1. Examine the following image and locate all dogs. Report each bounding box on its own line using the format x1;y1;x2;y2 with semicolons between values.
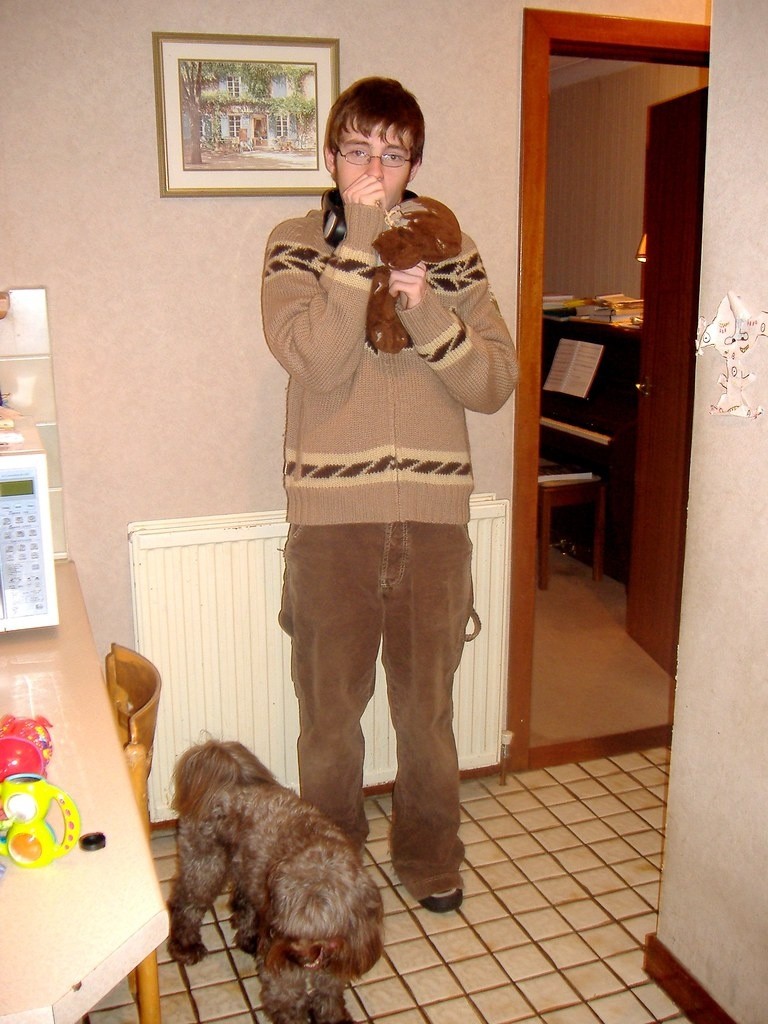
165;737;384;1024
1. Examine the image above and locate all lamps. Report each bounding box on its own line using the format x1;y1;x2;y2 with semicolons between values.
635;233;647;263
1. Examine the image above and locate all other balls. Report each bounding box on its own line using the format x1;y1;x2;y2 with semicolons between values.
0;719;53;784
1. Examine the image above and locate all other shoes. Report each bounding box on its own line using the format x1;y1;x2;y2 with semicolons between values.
421;887;461;911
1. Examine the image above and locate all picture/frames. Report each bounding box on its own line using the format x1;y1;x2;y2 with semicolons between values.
152;30;341;198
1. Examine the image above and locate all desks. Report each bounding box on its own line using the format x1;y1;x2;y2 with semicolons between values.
0;558;172;1024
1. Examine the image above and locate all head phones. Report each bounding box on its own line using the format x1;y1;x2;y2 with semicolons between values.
322;190;418;247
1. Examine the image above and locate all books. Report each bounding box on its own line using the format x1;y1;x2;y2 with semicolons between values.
543;338;603;398
543;293;645;323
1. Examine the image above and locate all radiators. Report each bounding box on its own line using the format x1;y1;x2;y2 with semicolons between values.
127;494;509;827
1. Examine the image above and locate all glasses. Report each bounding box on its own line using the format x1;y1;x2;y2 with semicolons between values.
336;147;416;166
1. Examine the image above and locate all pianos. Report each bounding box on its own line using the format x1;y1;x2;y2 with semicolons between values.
541;317;641;585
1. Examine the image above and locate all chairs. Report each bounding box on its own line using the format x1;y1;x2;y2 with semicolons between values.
104;641;163;1024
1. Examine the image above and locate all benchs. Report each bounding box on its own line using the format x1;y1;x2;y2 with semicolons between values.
538;457;605;590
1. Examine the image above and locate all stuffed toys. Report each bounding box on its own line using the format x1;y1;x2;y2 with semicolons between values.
367;196;463;354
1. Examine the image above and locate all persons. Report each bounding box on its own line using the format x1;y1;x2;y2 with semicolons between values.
261;77;518;912
